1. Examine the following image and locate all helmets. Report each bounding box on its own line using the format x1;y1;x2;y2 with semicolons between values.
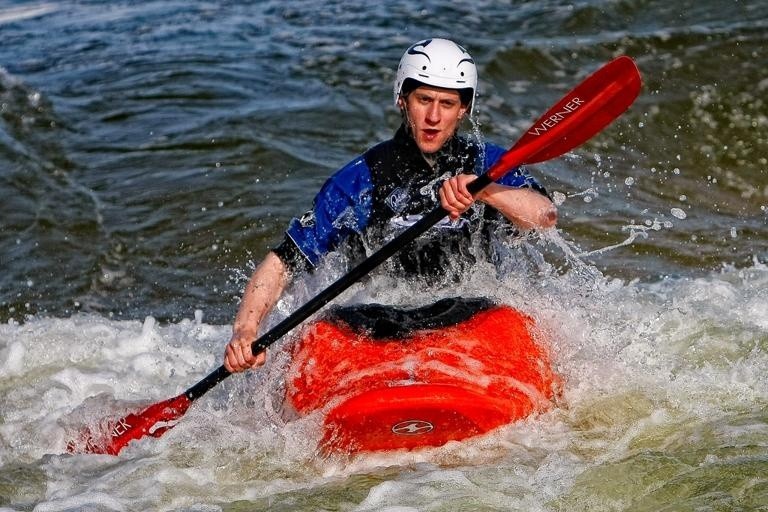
395;38;478;117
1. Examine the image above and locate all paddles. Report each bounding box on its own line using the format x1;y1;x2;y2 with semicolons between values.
67;53;642;455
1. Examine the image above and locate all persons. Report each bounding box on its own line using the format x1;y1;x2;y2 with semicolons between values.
223;37;562;377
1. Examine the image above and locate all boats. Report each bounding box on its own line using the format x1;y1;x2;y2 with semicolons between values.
282;295;566;463
